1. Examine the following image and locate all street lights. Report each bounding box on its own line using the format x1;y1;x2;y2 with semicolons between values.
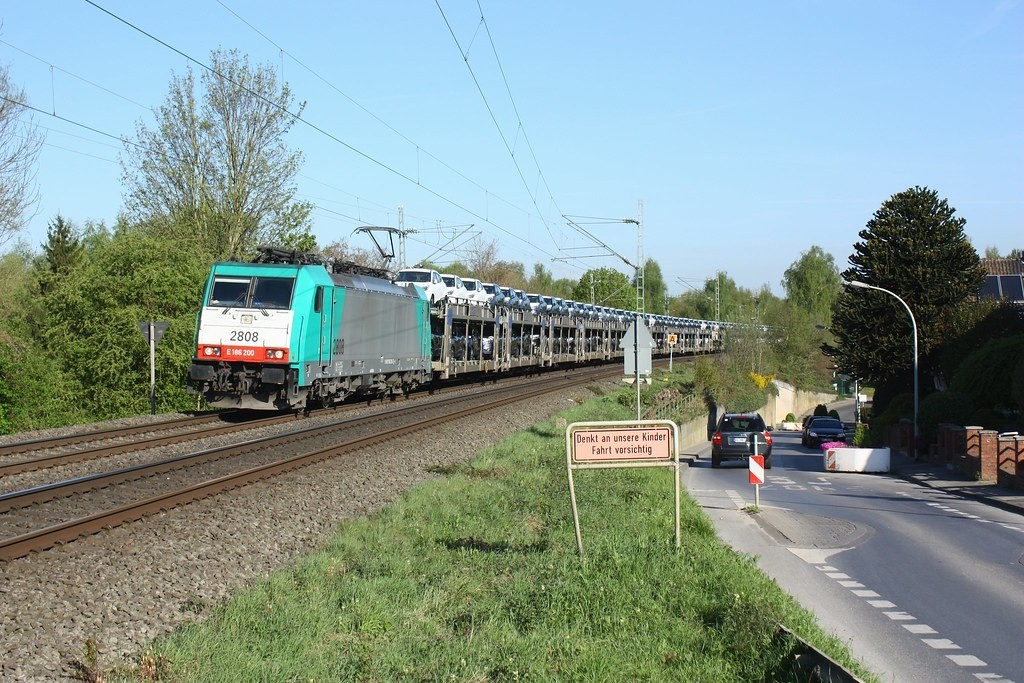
849;280;918;462
815;324;860;424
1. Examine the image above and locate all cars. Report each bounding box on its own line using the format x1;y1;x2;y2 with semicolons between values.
801;416;834;445
805;419;846;449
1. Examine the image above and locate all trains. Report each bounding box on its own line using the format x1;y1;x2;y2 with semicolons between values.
190;246;781;415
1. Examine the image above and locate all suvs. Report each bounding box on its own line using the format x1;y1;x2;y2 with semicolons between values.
709;412;773;469
